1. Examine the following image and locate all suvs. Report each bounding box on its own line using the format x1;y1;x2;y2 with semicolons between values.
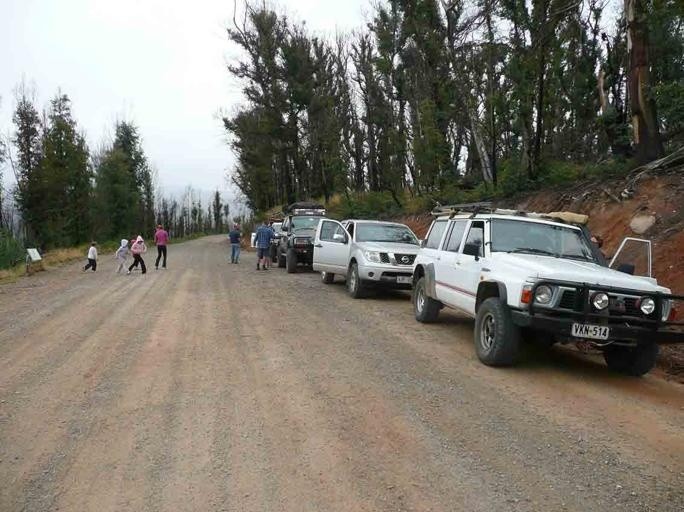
412;202;684;376
267;214;331;272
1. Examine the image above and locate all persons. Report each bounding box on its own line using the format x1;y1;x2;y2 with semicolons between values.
253;220;281;270
115;235;147;274
153;225;169;270
83;241;99;272
590;234;604;247
228;223;242;264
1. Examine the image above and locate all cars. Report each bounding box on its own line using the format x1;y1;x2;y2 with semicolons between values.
313;219;420;300
